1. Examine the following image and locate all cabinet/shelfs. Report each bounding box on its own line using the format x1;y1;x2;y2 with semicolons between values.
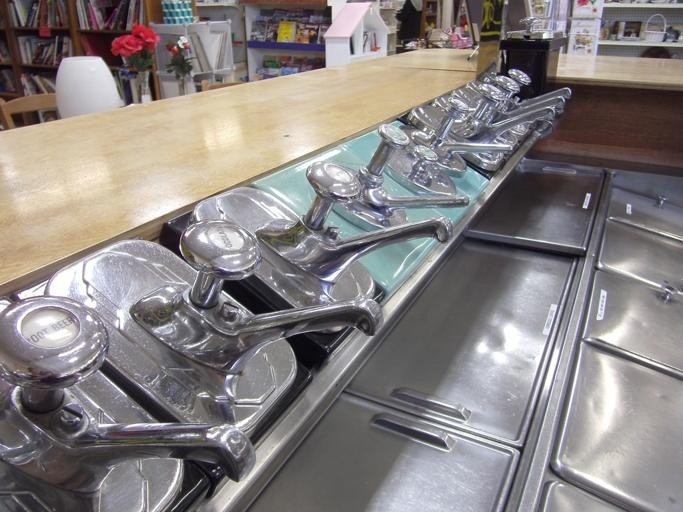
596;0;683;59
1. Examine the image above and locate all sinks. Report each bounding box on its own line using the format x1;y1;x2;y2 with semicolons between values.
506;29;564;38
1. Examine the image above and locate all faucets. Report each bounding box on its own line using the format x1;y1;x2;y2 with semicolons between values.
391;70;572;207
519;15;551;32
192;161;454;364
2;296;255;511
41;218;384;482
321;123;471;243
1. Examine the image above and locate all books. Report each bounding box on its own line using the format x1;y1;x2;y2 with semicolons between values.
253;53;323;79
249;7;329;44
0;0;145;123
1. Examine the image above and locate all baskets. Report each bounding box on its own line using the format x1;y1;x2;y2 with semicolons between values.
645;14;667;44
